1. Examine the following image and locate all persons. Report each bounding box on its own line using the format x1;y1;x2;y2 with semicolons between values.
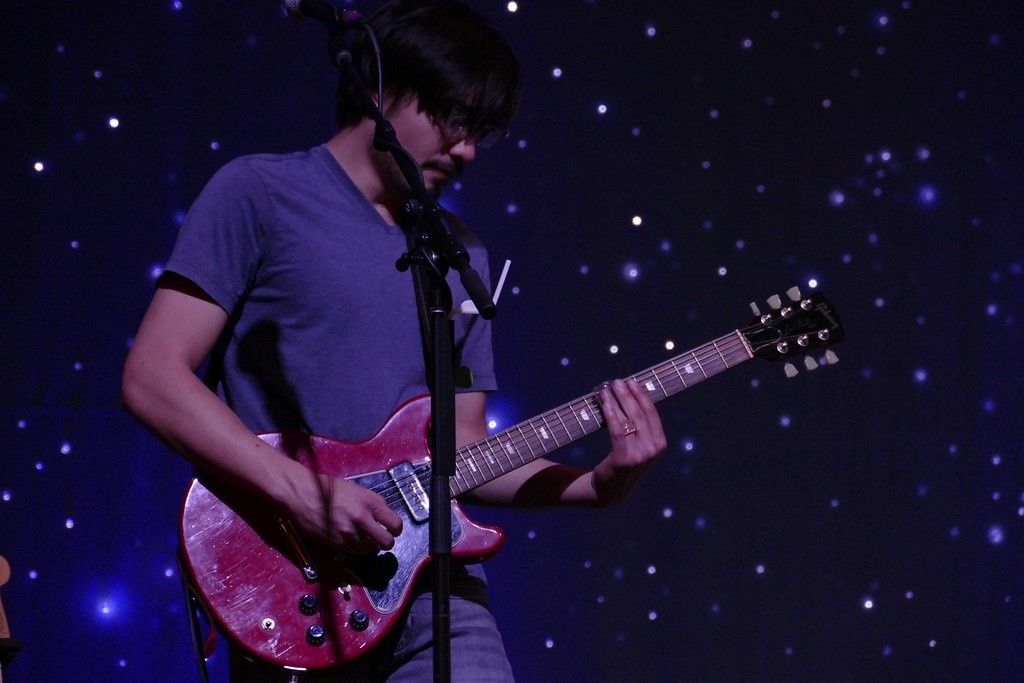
120;0;671;682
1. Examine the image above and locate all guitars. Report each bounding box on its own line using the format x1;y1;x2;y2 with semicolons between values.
168;283;858;678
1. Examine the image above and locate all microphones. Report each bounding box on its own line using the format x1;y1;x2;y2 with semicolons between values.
284;0;371;29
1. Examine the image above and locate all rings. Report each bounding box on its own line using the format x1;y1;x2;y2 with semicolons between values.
623;416;637;436
